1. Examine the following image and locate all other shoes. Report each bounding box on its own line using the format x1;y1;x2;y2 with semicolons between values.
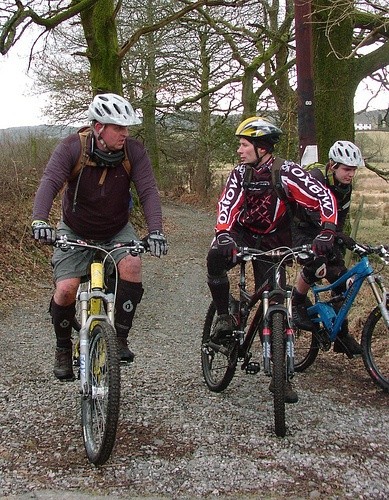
333;333;363;354
212;314;234;339
291;296;314;331
268;375;299;404
53;345;74;380
115;337;135;364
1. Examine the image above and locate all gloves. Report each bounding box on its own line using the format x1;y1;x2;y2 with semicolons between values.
146;230;168;258
214;233;239;264
29;220;56;247
334;232;356;251
311;230;335;256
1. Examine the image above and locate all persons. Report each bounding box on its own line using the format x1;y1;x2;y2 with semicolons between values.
203;117;337;404
32;93;170;380
278;138;369;355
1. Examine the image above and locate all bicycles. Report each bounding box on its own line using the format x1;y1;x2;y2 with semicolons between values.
258;238;389;393
201;243;318;438
32;233;171;464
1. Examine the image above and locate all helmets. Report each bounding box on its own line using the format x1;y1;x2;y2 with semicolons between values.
329;140;365;168
87;92;142;127
234;116;283;144
